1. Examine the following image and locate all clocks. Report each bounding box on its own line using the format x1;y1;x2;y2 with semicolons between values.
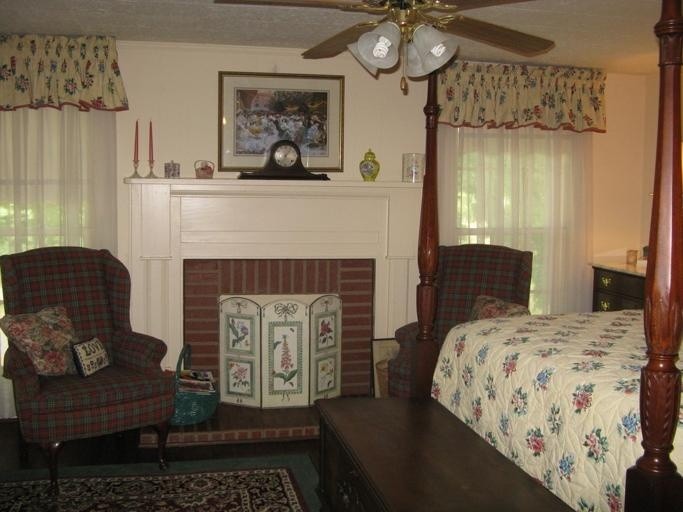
238;141;331;179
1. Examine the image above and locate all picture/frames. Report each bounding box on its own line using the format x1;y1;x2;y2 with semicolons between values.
217;70;345;175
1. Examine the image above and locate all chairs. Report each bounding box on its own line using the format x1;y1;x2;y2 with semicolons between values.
385;241;534;400
0;246;179;511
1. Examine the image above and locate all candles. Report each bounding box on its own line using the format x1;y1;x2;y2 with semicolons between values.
133;118;139;161
147;120;154;160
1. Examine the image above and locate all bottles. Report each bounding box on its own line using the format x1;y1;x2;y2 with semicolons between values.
360;147;379;182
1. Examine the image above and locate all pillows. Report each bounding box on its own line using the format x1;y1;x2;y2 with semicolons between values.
0;307;78;378
70;335;112;378
471;293;530;321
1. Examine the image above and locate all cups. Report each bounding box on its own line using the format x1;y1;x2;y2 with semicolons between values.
163;160;180;178
625;264;636;273
402;152;425;182
625;250;638;265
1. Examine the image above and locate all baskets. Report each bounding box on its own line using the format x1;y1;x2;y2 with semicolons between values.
169;344;219;426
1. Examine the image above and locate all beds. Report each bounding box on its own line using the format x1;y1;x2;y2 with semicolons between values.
411;0;683;512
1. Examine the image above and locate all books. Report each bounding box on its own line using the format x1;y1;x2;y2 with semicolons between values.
179;370;215;393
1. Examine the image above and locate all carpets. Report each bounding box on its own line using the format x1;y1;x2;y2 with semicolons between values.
4;450;318;512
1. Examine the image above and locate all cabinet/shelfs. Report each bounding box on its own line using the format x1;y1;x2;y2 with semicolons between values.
590;255;647;310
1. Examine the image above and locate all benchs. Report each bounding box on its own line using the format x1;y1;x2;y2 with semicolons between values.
313;396;580;511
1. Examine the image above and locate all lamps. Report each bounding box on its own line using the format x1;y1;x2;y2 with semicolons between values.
347;21;460;81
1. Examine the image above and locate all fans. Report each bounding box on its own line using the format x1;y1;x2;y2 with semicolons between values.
210;0;556;59
